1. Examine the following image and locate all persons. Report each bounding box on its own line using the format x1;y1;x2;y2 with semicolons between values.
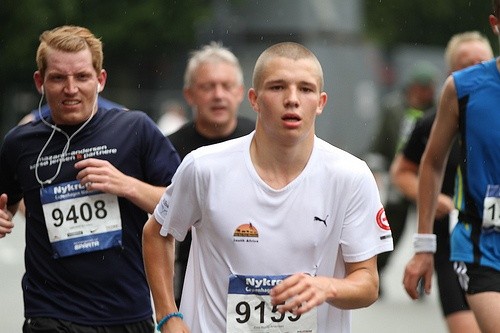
141;42;394;333
0;26;256;333
371;7;500;333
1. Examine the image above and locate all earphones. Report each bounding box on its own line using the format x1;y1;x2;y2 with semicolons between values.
40;84;44;95
97;82;101;93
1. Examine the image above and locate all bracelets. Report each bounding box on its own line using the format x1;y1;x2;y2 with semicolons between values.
413;234;437;253
156;313;184;330
